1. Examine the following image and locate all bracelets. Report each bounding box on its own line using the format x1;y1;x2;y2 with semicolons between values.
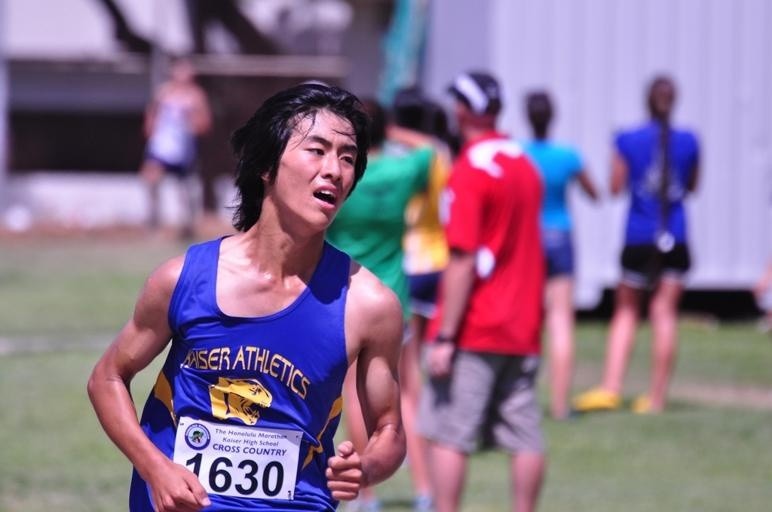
433;333;458;344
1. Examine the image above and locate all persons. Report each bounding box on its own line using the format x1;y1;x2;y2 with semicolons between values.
319;69;469;510
139;54;210;243
570;75;701;415
420;65;553;511
510;88;601;421
88;77;410;511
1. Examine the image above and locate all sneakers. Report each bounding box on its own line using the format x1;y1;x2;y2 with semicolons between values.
632;396;652;414
570;390;618;414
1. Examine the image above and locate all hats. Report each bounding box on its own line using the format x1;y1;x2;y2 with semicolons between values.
448;73;500;113
